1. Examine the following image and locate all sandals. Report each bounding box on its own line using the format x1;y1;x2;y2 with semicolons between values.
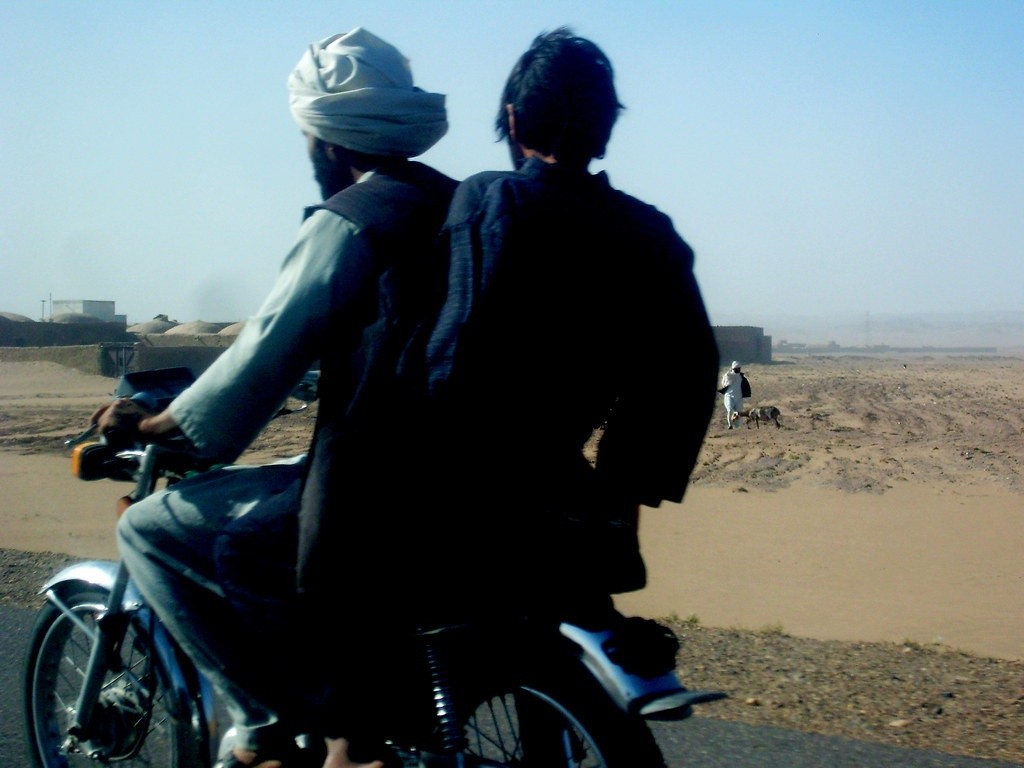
212;745;287;767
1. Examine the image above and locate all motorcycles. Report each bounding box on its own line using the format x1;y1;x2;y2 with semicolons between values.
22;364;727;768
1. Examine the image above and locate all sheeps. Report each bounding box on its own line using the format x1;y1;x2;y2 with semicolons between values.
731;405;782;429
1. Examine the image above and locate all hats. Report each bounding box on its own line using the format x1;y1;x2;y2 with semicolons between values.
732;360;742;369
286;27;448;158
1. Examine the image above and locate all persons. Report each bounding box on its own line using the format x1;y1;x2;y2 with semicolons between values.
722;360;743;429
96;25;723;768
